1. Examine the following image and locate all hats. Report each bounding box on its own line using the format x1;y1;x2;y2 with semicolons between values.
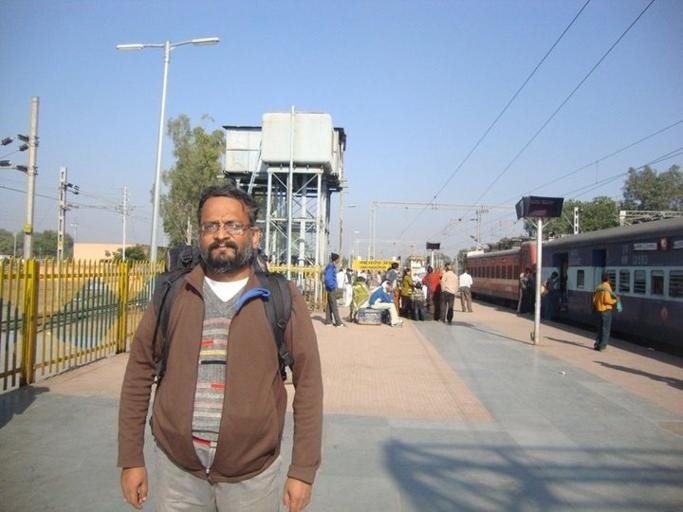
331;253;339;262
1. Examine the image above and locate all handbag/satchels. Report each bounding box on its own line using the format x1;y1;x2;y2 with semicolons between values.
540;286;548;295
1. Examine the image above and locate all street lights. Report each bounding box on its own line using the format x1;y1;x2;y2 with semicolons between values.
69;221;78;240
112;33;221;262
338;203;357;265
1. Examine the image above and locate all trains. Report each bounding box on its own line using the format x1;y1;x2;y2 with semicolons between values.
458;215;682;345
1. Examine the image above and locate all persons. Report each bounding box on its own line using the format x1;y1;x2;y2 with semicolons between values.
117;183;323;512
322;252;474;330
516;266;568;322
590;273;623;354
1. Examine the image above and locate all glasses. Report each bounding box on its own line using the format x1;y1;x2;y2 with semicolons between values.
200;223;252;235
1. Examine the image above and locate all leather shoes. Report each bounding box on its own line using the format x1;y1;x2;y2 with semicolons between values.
600;348;611;353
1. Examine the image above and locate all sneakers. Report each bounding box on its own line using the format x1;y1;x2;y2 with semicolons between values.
392;320;403;326
336;323;347;328
